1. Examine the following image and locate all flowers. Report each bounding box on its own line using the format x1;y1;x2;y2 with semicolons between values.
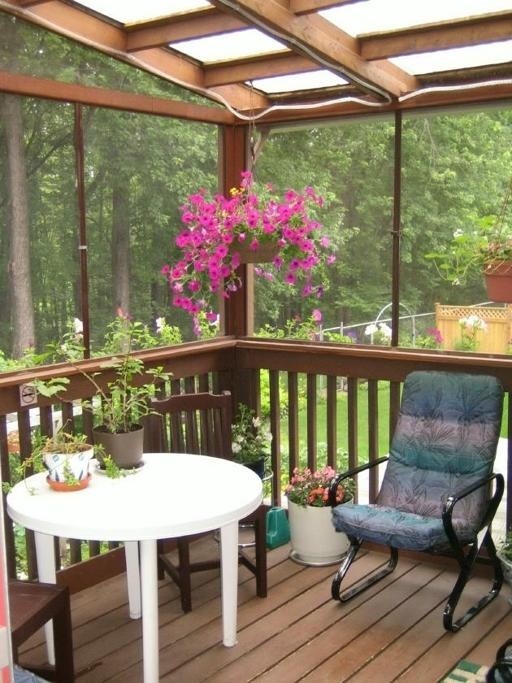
225;404;273;461
58;302;180;362
162;169;336;340
425;220;512;286
418;325;443;346
285;465;355;507
451;313;487;351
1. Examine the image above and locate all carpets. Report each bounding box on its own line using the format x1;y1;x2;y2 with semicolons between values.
444;661;490;683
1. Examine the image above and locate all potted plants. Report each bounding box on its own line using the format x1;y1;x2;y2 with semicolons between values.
1;418;126;497
33;320;173;467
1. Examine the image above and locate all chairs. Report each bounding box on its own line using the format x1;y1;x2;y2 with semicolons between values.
4;493;76;683
149;391;270;614
6;453;263;683
328;371;505;634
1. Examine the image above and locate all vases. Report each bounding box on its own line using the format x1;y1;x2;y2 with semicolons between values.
288;496;354;561
225;455;264;481
228;238;282;263
483;260;512;304
